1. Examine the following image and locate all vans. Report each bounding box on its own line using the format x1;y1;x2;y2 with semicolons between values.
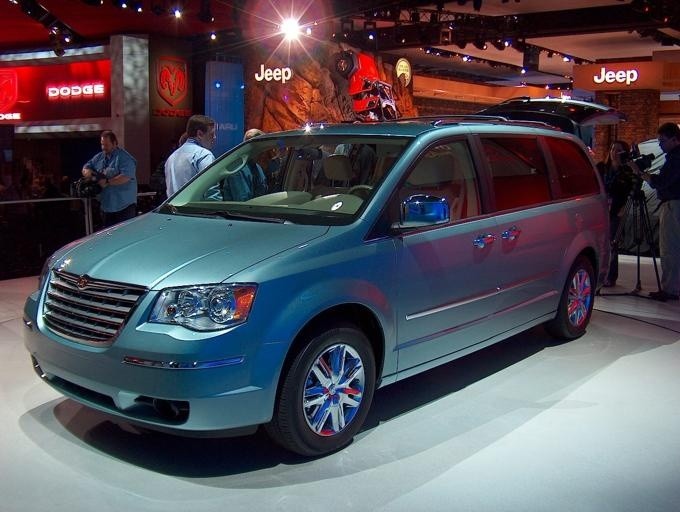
19;94;631;457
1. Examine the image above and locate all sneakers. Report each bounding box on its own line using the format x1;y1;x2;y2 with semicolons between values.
604;279;616;287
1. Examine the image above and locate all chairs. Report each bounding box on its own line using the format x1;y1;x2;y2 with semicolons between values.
315;154;404;196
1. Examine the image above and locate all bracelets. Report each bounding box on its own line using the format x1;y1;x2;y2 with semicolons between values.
106;178;110;183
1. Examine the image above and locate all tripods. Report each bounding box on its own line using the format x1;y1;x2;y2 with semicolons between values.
82;198;95;235
596;180;665;302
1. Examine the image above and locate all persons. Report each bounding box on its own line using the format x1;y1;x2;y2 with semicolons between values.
596;123;680;300
81;131;137;228
150;114;378;209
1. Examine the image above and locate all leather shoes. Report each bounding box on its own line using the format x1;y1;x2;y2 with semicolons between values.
649;291;679;302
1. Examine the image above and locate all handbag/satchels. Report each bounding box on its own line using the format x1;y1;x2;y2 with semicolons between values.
70;175;109;198
